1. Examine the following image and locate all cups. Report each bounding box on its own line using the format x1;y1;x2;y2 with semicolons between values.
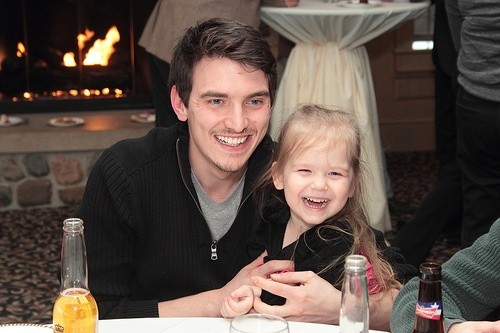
229;313;289;333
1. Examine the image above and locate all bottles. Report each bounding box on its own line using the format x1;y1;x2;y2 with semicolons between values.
413;262;444;333
52;218;99;332
339;255;369;333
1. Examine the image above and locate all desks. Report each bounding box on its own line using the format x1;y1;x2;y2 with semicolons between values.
257;1;431;232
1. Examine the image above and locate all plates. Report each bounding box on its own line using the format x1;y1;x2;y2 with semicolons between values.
48;117;84;126
1;116;22;127
131;112;156;123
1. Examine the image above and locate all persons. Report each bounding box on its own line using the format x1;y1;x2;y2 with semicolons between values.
389;217;500;333
137;0;263;127
221;106;404;318
81;18;421;333
456;0;500;249
